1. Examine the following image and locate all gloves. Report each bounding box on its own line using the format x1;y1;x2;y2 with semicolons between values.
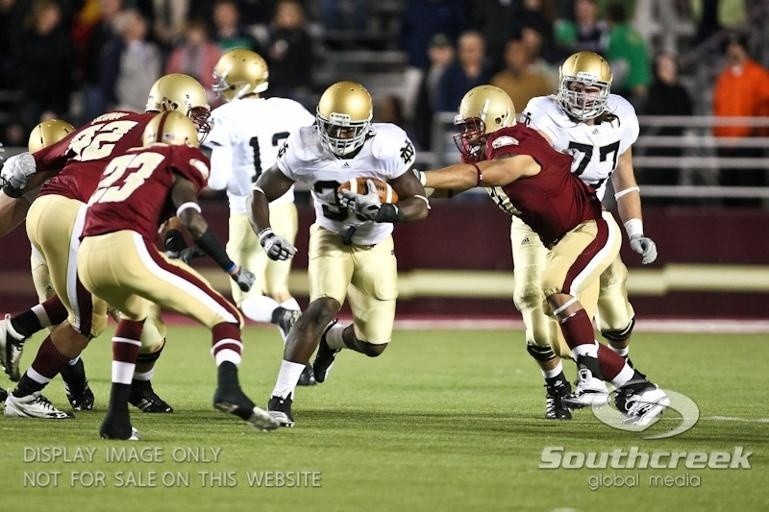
232;264;256;291
258;228;297;261
1;153;36;191
338;180;387;223
628;233;658;264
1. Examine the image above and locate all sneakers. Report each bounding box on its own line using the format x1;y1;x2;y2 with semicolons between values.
213;381;279;430
131;380;172;413
268;307;339;427
544;355;672;426
1;314;93;422
100;424;141;442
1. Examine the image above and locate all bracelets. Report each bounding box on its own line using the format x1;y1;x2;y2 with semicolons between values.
623;217;644;238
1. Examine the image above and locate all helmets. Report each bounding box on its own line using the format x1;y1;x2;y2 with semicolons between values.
452;84;516;143
558;51;613;106
316;81;373;151
27;119;74;154
211;48;268;101
143;73;209;146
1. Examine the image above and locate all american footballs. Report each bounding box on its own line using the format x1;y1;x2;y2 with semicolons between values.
336;176;400;207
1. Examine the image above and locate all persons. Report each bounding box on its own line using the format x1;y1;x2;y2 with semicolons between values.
0;75;211;420
510;50;657;421
245;80;430;427
75;110;280;441
0;119;173;414
197;50;316;385
408;86;669;421
1;0;769;210
